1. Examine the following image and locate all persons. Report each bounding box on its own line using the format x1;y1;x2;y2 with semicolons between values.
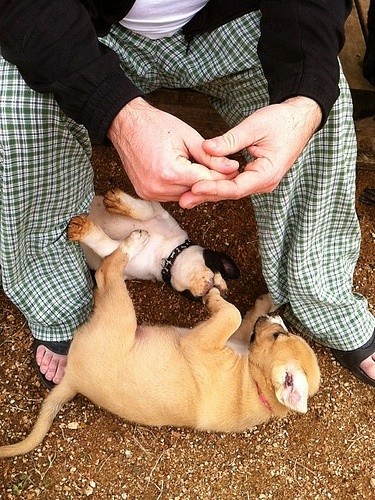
1;1;375;386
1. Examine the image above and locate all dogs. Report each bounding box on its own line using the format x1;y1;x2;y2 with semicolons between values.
1;230;321;459
68;186;242;305
